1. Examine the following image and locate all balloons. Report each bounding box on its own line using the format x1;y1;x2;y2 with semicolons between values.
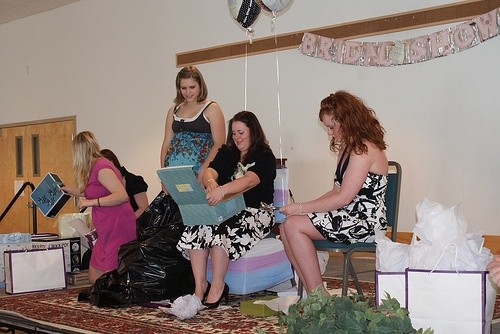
228;0;290;28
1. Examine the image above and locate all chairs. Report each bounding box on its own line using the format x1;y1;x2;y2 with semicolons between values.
297;161;402;304
206;158;297;299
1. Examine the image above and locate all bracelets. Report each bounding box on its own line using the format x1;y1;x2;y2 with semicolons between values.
97;198;101;207
205;179;215;185
299;202;304;215
220;185;225;197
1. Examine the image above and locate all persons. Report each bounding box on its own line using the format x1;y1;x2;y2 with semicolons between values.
100;149;148;219
487;255;500;287
279;92;389;313
176;111;277;308
56;132;136;284
161;66;226;196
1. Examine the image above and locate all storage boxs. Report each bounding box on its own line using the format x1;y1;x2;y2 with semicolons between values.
30;174;70;219
157;165;245;226
32;237;82;274
241;297;280;317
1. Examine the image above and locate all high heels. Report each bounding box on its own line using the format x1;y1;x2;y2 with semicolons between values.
199;281;211;303
203;282;229;310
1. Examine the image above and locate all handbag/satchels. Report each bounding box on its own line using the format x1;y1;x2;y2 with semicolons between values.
374;269;406;311
404;242;498;334
3;246;66;294
